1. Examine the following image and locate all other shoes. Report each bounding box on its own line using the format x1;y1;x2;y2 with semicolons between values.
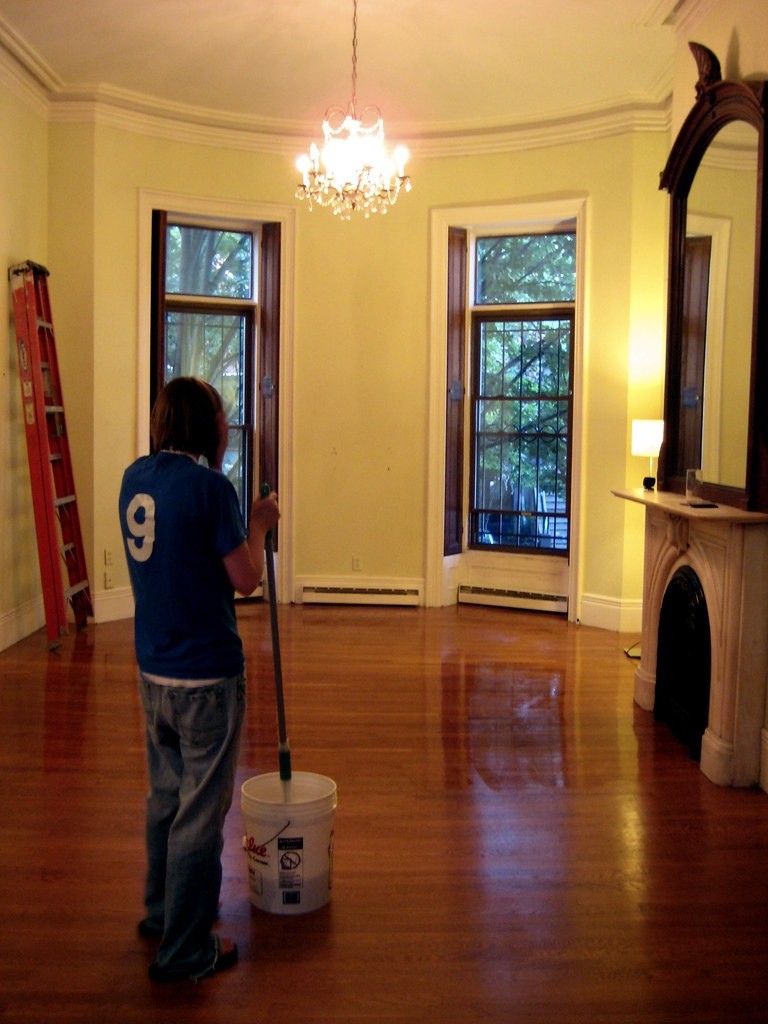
214;938;239;975
138;920;159;939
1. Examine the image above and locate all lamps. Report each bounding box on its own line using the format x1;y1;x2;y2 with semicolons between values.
631;420;664;490
296;0;412;222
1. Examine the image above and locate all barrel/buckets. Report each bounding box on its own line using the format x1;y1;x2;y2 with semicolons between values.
239;770;338;915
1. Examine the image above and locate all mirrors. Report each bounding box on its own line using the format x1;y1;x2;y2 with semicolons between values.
656;40;768;511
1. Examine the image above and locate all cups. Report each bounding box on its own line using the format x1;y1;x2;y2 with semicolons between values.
686;469;703;505
643;477;655;490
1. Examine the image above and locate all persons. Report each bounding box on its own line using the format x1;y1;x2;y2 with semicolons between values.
117;377;283;987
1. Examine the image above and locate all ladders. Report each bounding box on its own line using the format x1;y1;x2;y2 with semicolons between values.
9;259;97;651
